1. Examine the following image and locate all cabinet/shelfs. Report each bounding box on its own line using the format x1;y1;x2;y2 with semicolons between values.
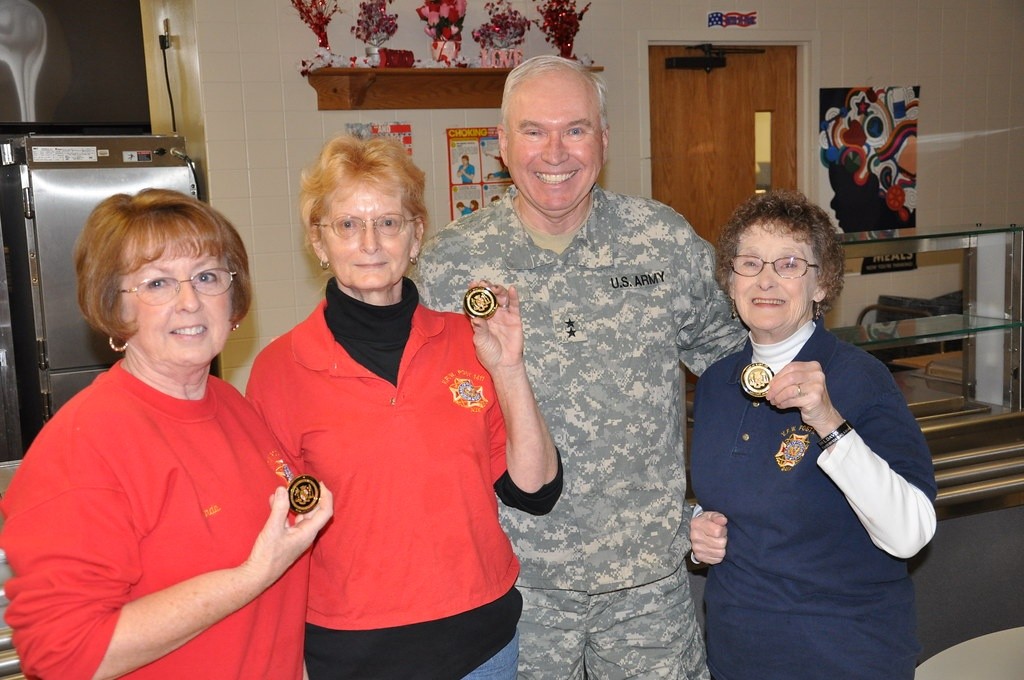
827;224;1023;420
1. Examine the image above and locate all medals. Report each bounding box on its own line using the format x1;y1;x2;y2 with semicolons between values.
741;362;775;398
287;474;320;514
464;287;498;322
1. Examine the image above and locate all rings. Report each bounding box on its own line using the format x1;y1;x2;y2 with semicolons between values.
497;304;508;308
798;384;801;395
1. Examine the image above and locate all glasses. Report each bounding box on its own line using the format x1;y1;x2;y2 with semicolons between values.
313;214;420;239
729;255;820;280
118;268;236;306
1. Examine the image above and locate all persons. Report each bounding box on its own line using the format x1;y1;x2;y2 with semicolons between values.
406;56;748;680
244;137;564;679
1;188;334;680
686;193;938;680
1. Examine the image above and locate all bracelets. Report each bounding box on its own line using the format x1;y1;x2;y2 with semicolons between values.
816;420;854;451
